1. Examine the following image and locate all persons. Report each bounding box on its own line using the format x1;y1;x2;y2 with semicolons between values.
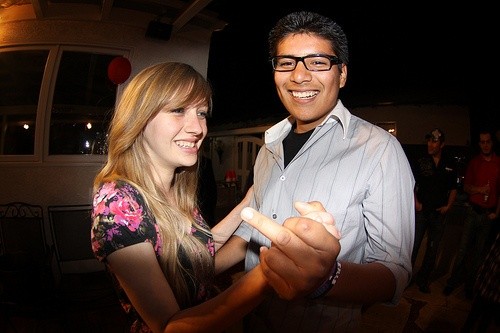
411;128;458;293
442;129;500;299
212;11;416;333
90;62;343;333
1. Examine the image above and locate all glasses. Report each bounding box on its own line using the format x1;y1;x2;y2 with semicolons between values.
269;53;341;72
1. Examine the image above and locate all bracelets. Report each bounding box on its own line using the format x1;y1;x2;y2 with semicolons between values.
316;262;341;296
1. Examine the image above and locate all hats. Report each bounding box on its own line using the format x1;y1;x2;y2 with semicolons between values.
425;127;446;142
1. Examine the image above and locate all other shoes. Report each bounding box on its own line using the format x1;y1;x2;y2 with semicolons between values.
415;273;430;294
443;284;454;296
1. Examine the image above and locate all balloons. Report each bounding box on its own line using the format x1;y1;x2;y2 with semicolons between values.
107;57;132;85
